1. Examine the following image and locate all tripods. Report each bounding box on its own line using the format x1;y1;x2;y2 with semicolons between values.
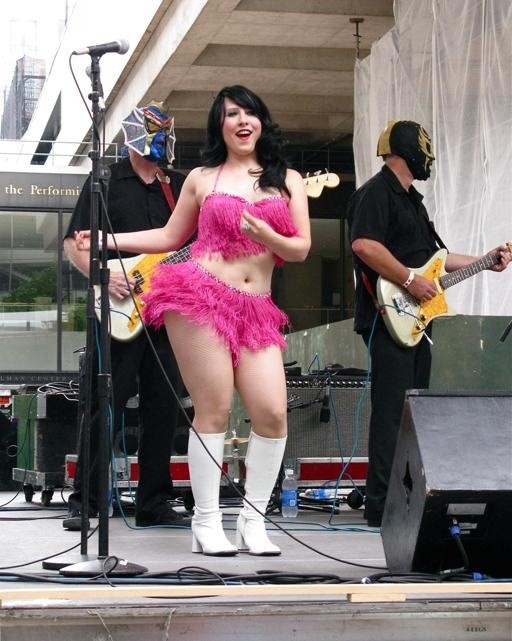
265;461;340;517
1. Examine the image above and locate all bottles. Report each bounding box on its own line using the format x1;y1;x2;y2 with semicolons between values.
313;489;332;498
97;471;114;517
281;469;299;518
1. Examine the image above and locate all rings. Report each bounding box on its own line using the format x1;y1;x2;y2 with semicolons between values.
242;223;249;232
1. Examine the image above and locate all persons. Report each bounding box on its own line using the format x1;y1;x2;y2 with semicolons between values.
73;85;312;556
344;122;511;527
63;105;192;529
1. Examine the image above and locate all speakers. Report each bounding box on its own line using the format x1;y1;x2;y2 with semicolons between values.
281;375;372;458
380;386;512;580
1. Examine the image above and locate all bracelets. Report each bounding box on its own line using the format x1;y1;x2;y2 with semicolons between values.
402;271;415;288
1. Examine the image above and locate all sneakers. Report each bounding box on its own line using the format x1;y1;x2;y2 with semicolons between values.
63;510;89;531
136;507;192;526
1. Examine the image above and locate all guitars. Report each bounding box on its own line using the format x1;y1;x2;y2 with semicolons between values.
377;240;512;348
94;168;341;343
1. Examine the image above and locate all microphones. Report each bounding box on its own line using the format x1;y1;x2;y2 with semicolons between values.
85;66;106;112
72;38;130;55
320;378;331;423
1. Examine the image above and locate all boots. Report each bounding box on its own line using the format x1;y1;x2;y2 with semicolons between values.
236;428;287;556
187;428;238;555
